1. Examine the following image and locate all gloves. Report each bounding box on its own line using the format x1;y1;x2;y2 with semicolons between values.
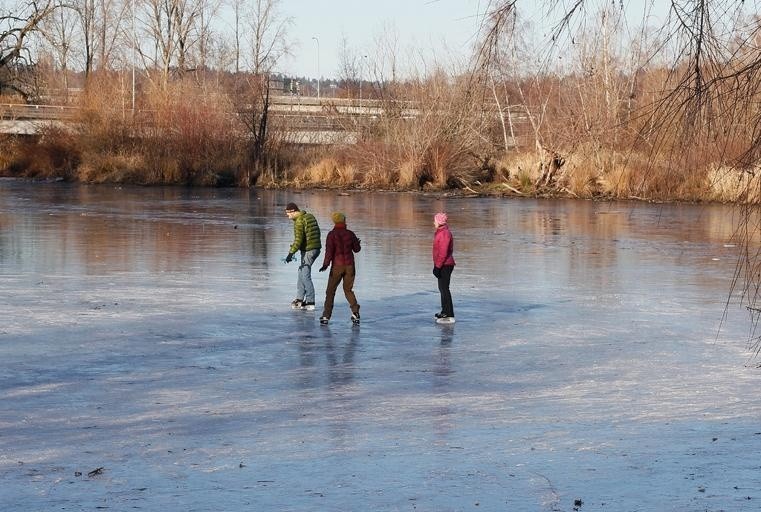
433;268;440;278
319;267;326;272
285;253;293;263
357;238;361;243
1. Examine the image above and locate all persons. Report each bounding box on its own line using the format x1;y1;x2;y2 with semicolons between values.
282;201;323;307
436;325;455;421
322;325;364;430
290;307;318;398
431;211;457;319
319;212;363;321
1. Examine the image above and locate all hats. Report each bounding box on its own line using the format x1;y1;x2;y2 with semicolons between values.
434;212;448;225
331;212;346;223
285;203;299;211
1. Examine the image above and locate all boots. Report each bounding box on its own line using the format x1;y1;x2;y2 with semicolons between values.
351;312;361;327
292;299;315;310
319;316;328;326
434;311;455;324
